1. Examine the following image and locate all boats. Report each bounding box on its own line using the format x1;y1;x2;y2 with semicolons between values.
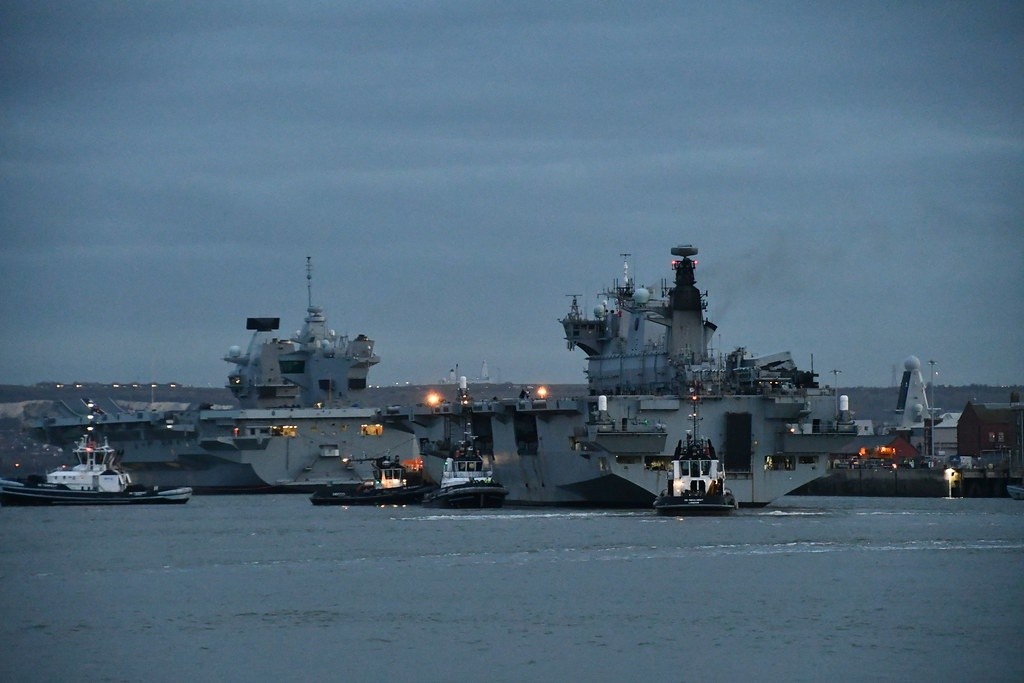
0;402;193;506
18;255;420;493
1007;485;1024;500
308;448;426;506
364;244;898;510
421;418;510;508
653;386;739;516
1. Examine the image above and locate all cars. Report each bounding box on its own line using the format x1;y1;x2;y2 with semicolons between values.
926;456;932;462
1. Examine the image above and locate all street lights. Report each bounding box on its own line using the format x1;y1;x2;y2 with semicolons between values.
828;369;844;428
928;360;939;464
